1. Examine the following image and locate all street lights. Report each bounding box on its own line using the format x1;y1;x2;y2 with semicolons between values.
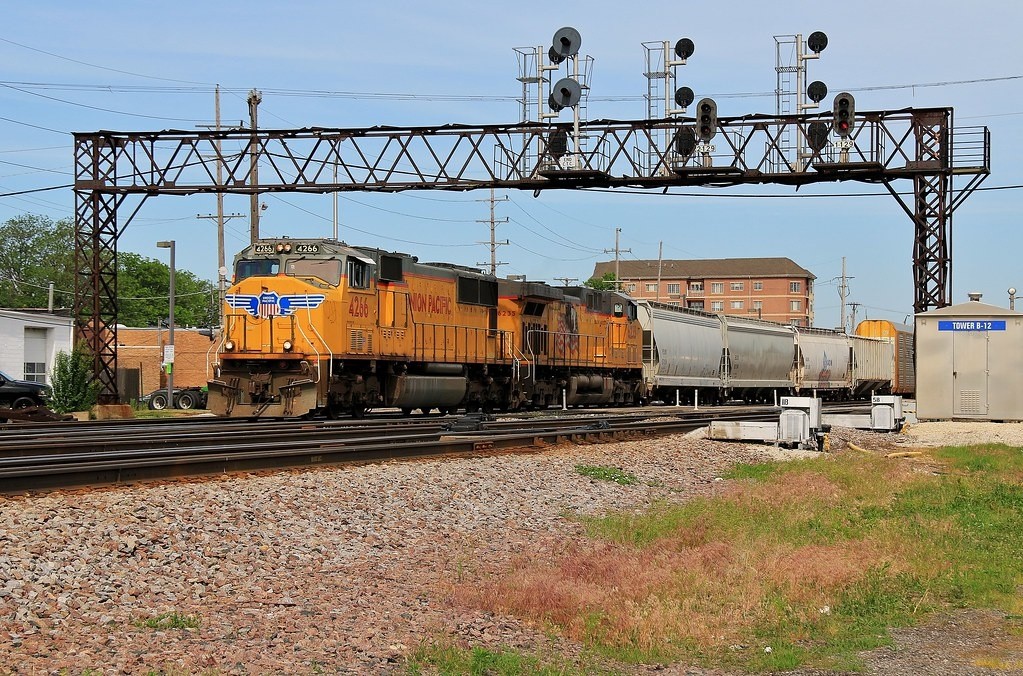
156;239;176;410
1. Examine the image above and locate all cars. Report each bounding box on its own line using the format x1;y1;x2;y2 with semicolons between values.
0;369;53;423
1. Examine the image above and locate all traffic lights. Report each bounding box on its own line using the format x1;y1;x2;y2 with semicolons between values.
833;92;855;136
696;98;718;140
553;27;582;56
552;77;582;107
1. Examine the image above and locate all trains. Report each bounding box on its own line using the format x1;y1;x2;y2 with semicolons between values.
205;231;915;419
